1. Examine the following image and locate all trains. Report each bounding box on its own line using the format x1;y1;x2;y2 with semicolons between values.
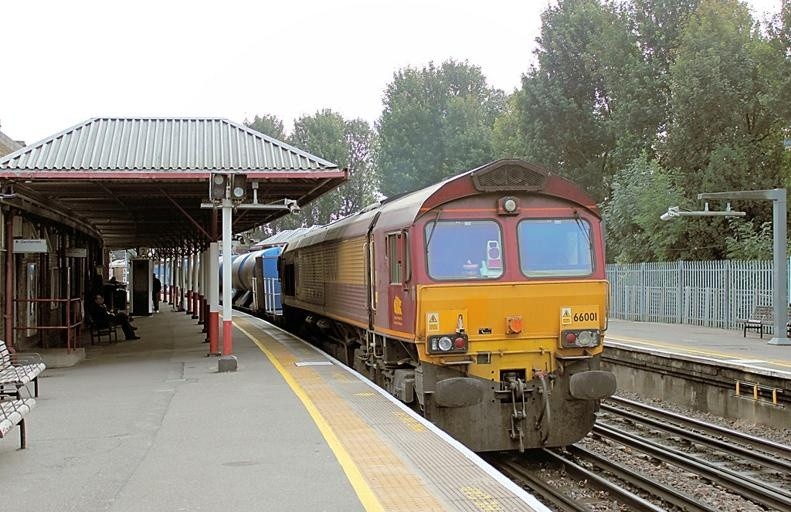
156;158;618;454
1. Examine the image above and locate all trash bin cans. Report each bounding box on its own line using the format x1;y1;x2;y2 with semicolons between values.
113;290;126;310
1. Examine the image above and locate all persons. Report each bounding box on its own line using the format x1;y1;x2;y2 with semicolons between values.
152;273;162;313
100;294;127;342
93;293;141;340
110;276;120;283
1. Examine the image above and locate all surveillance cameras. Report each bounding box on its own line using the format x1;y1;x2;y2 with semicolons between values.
660;211;674;221
290;206;301;215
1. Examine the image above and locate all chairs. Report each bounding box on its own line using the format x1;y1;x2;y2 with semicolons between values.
735;305;791;339
85;314;117;345
0;340;47;449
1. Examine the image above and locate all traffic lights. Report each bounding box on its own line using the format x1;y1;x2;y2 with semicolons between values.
209;172;248;203
